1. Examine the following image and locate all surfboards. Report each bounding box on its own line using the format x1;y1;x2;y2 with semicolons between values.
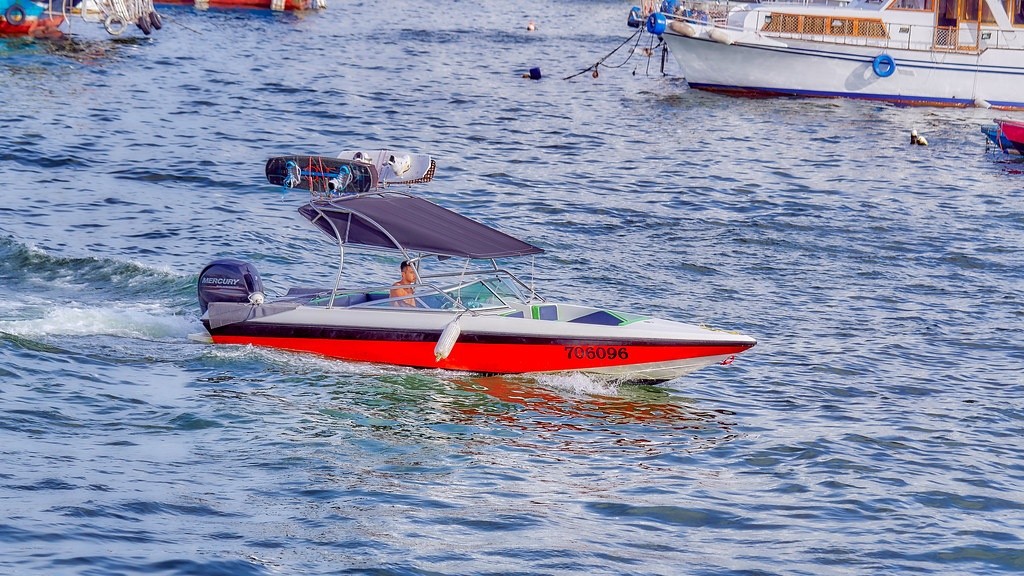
337;149;436;184
266;154;378;194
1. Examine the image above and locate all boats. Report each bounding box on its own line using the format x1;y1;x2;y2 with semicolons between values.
629;0;1024;110
265;156;380;194
198;187;757;386
981;124;1021;155
993;118;1024;156
0;0;45;39
337;150;436;184
56;16;155;43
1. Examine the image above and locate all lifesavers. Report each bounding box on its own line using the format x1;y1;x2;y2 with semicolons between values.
6;4;26;26
104;14;128;36
138;16;152;35
873;54;896;77
149;11;162;30
627;7;641;29
645;13;667;36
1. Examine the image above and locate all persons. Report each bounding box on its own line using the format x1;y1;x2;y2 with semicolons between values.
910;130;917;144
675;6;698;23
390;261;417;307
